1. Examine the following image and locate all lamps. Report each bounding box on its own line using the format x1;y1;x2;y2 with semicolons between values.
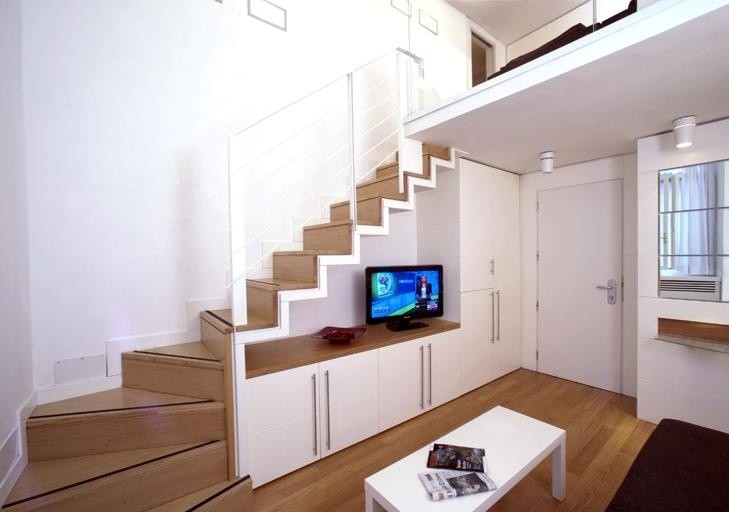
672;117;696;149
540;151;555;173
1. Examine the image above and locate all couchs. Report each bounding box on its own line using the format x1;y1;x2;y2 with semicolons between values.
602;418;729;512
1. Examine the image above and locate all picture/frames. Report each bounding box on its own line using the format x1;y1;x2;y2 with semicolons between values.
419;9;438;34
248;0;286;31
390;0;414;18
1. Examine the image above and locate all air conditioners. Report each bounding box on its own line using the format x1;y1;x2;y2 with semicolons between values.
659;276;721;302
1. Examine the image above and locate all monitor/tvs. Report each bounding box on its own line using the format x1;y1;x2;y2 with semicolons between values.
365;264;444;332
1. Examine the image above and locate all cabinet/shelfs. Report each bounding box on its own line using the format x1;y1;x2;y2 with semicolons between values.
379;318;461;433
246;324;379;490
417;159;519;396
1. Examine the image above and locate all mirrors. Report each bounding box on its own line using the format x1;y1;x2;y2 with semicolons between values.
657;159;728;305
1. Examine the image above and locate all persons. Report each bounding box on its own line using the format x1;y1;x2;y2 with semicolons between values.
415;275;433;306
